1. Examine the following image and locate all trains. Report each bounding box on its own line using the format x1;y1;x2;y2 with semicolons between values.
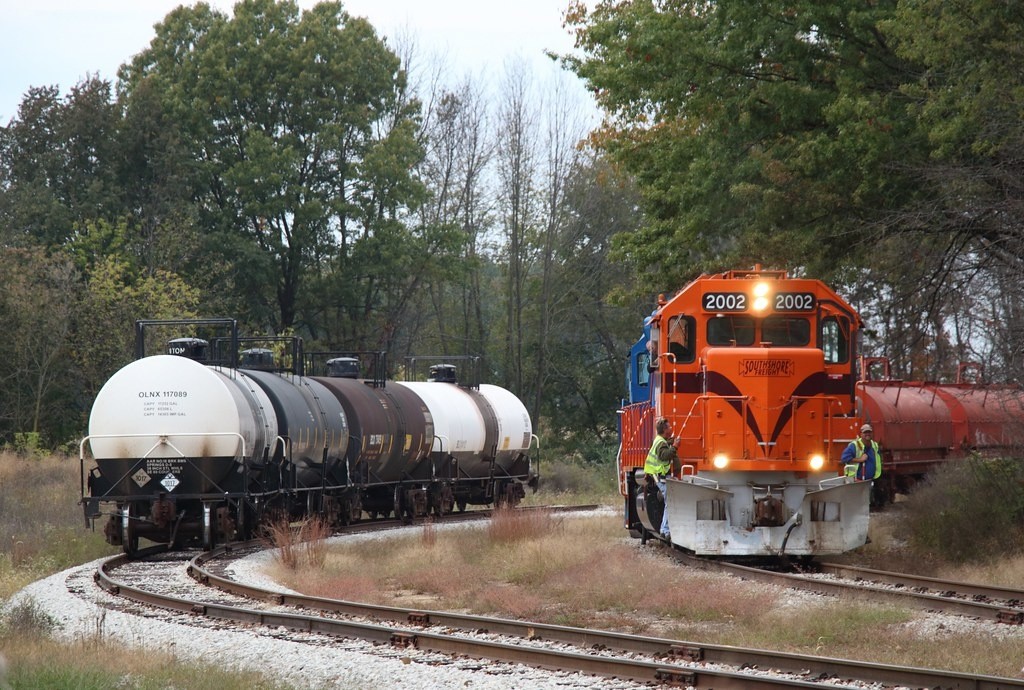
615;261;1023;573
77;316;542;562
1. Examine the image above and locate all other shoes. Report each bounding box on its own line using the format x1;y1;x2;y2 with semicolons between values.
660;532;670;540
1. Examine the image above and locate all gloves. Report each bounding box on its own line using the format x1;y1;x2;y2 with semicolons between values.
672;438;681;450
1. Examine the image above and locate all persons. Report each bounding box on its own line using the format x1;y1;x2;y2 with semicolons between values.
643;419;682;534
841;425;882;544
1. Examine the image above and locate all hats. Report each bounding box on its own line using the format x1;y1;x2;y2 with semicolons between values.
860;424;872;432
